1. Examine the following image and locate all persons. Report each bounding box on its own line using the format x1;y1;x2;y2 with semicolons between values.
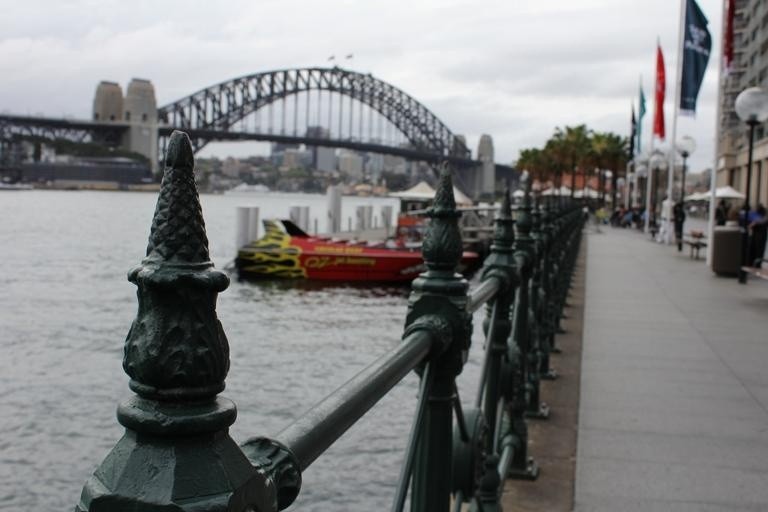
582;194;768;269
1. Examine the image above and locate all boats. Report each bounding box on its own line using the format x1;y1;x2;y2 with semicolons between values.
235;212;495;289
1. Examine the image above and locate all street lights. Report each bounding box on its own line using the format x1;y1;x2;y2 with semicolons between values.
734;86;767;284
604;136;695;249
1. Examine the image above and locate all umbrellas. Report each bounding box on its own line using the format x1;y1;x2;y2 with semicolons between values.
707;185;746;200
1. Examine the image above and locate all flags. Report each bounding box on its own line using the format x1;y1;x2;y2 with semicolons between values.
654;45;666;138
630;110;637;160
676;0;712;118
637;86;646;154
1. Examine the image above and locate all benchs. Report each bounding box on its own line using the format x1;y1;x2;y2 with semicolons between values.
741;258;768;282
675;230;706;260
